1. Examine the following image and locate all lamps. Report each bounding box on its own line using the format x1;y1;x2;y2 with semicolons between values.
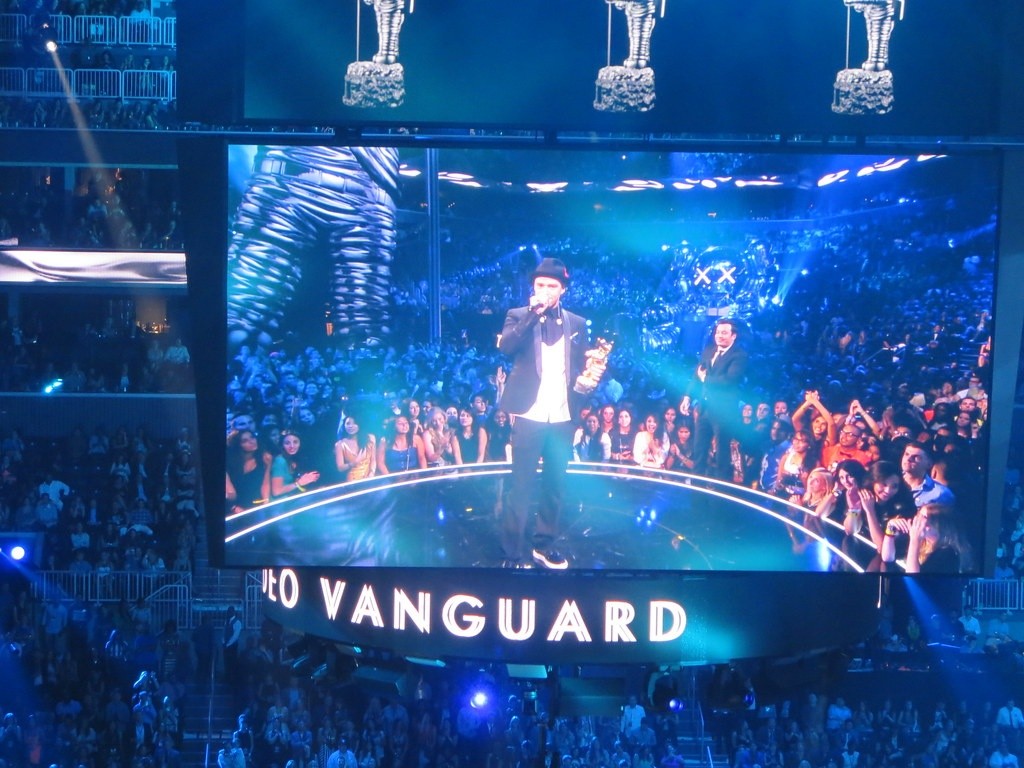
287;635;854;718
0;541;30;567
31;11;58;53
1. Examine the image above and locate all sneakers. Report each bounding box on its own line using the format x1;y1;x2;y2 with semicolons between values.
504;546;570;568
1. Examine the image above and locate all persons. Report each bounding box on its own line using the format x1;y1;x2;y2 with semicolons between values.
0;166;200;768
226;338;513;517
191;590;1024;768
227;150;994;339
995;485;1024;580
573;340;992;573
0;0;176;131
499;258;607;570
679;319;744;482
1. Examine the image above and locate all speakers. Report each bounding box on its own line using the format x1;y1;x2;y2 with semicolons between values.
350;664;411;700
548;678;625;717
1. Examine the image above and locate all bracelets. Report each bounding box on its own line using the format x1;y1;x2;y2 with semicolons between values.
616;453;620;460
294;481;307;493
885;525;896;538
846;508;861;517
831;487;843;498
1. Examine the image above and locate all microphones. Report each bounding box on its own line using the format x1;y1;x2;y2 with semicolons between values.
529;302;544;312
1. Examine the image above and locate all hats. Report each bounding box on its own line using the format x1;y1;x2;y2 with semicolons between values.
532;258;568;289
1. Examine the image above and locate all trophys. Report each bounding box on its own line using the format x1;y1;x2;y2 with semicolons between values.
573;338;614;395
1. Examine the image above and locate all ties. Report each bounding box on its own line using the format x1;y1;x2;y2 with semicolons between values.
713;350;723;366
